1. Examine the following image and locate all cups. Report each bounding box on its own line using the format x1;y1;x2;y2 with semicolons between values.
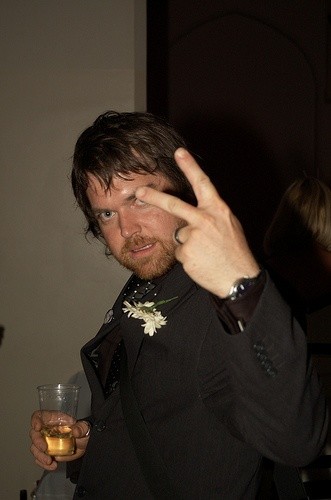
36;383;81;456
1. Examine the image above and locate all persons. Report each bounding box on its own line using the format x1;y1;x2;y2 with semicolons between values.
261;174;331;500
29;112;330;498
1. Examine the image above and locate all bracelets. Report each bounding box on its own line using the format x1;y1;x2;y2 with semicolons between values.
82;420;91;437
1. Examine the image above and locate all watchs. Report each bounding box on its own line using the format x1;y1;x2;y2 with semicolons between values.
211;270;267;303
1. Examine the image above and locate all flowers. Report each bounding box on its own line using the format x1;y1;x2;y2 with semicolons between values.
121;295;179;337
